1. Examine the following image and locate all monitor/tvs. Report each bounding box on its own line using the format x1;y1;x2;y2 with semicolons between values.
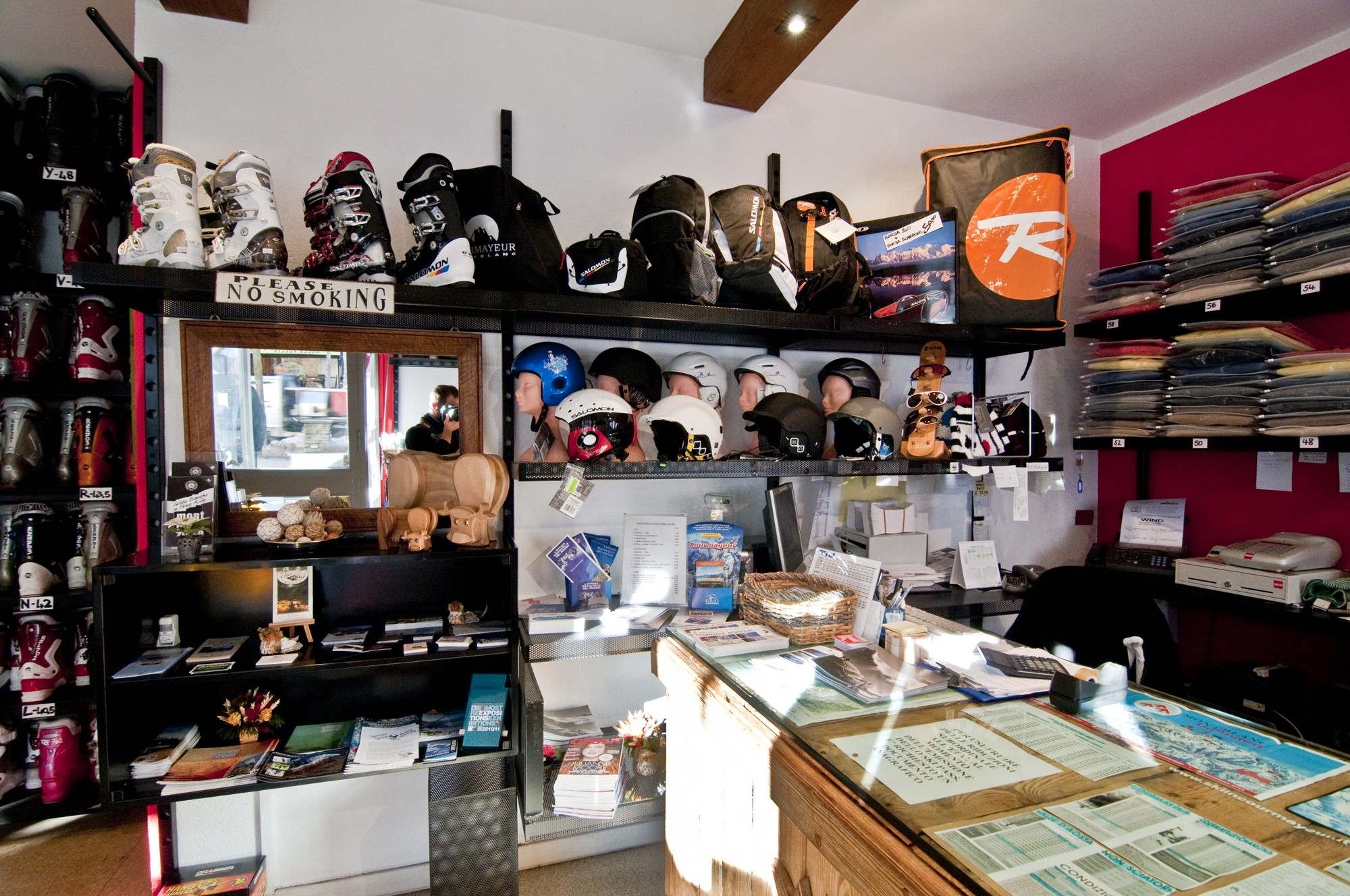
760;482;808;575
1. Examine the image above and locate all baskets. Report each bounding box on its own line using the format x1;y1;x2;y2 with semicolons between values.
737;572;857;645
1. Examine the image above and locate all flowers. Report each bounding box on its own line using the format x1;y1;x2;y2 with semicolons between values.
613;710;666;758
216;686;283;738
543;744;555;763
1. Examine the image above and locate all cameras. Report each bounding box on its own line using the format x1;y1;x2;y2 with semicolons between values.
440;404;459;423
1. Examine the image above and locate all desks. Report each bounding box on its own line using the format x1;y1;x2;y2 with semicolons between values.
650;605;1349;896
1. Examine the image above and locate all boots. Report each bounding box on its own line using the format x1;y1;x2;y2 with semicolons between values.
202;151;291;276
393;152;476;287
0;69;127;828
117;142;206;269
289;151;396;282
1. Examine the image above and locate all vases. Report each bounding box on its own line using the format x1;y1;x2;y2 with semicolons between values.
239;727;257;743
543;762;554;783
638;747;658;776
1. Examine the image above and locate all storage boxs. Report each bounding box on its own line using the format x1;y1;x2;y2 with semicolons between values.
158;855;267;896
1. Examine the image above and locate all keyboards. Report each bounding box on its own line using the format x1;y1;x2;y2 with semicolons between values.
878;599;979;637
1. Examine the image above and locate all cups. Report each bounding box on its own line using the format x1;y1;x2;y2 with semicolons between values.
880;606;907;637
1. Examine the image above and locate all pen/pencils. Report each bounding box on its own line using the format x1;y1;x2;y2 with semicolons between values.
875;578;914;609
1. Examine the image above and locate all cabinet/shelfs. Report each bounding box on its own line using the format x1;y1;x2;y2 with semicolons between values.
90;548;518;811
66;262;1065;844
1072;274;1350;636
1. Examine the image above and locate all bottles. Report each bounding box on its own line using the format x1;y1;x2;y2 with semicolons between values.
702;492;753;610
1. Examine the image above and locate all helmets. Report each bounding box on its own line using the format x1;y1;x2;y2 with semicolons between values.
662;351;727;410
554;388;637;462
734;354;799;396
817;357;881;400
588;347;663;403
510;342;587;406
742;392;827;460
826;397;902;459
639;395;723;460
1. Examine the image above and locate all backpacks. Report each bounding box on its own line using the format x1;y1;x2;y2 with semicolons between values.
629;174;723;306
782;191;861;311
707;185;800;311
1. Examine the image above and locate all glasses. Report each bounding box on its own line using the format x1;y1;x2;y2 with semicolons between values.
905;404;943;425
910;363;950;381
905;390;948;408
903;415;940;439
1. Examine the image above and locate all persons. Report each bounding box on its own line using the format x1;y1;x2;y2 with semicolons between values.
588;347;663;462
818;358;881;459
401;384;460;455
732;353;800;454
511;342;586;461
661;351;727;418
559;738;616;775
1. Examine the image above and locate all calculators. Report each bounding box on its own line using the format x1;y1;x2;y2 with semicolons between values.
977;645;1070;680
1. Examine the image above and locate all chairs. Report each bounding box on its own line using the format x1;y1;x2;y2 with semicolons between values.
1006;566;1181;694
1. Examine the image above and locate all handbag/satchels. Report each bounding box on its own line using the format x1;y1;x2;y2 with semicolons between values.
560;228;647;301
455;165;568;296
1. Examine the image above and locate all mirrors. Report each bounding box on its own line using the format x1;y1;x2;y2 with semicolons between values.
179;319;484;538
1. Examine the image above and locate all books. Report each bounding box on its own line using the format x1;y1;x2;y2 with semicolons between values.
112;636;252;679
129;712;467;796
593;603;789;657
320;616;509;657
813;642;954;706
543;705;629;819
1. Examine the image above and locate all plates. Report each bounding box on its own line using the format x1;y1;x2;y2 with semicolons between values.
264;533;344;548
